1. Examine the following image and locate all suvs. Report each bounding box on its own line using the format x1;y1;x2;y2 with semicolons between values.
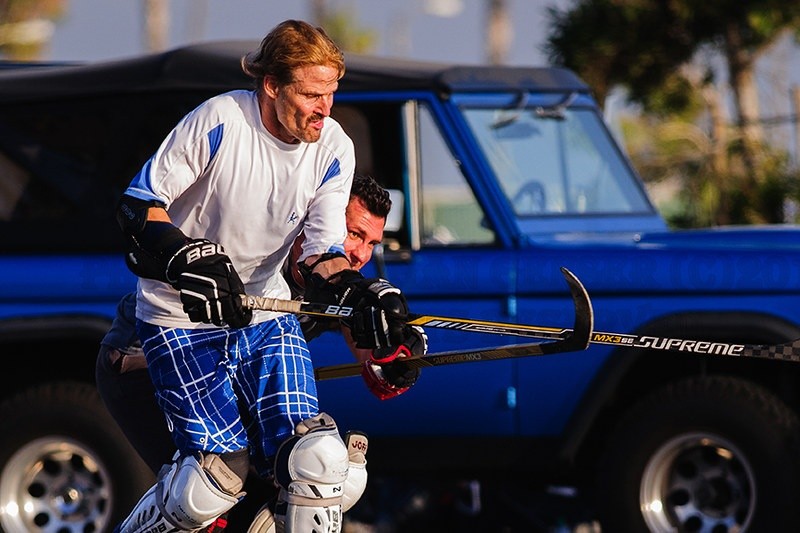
0;43;800;533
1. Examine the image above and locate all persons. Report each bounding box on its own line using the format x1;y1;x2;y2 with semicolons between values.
96;174;428;533
115;20;408;533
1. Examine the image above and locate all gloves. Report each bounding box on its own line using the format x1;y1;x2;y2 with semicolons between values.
331;276;409;349
362;324;429;400
163;238;253;328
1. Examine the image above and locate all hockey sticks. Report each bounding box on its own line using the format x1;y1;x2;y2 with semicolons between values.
241;294;800;361
314;266;594;381
